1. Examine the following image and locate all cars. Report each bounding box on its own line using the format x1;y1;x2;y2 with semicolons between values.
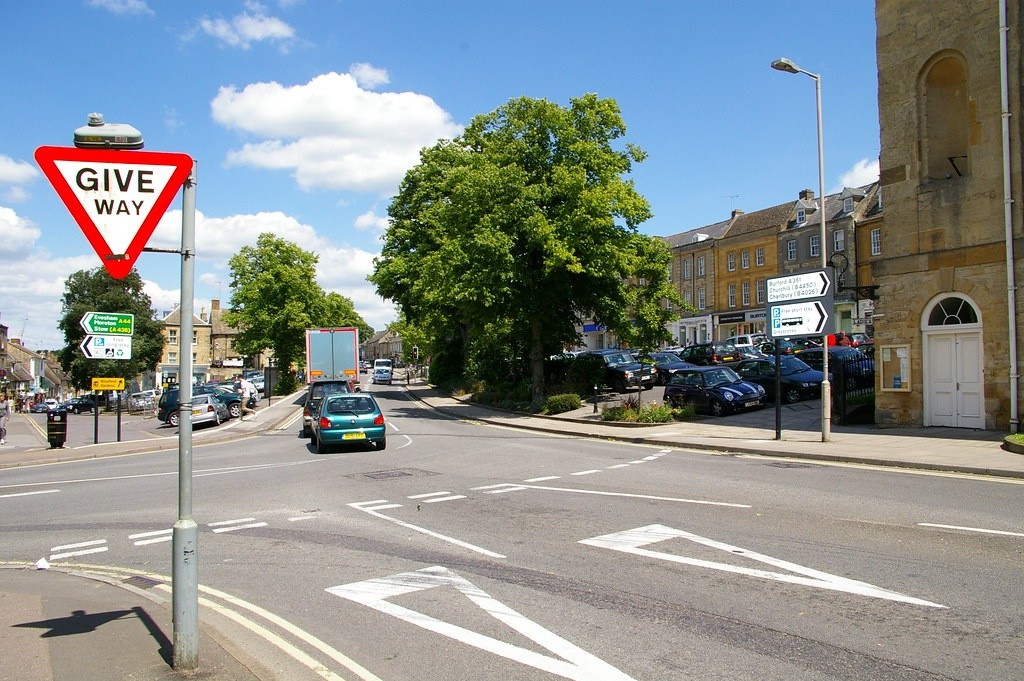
629;332;875;403
310;392;386;454
662;366;768;417
107;370;265;413
374;367;392;384
190;393;231;426
56;396;97;415
32;402;49;414
45;399;58;411
358;359;372;374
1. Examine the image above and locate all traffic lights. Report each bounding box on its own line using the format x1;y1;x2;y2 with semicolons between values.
413;347;419;359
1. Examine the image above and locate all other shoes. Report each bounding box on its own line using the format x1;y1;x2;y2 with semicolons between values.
235;419;241;422
252;410;256;415
1;439;4;444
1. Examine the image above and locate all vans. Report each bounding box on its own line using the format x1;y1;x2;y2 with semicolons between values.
373;359;394;374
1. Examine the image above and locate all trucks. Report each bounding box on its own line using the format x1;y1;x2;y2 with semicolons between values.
305;325;361;390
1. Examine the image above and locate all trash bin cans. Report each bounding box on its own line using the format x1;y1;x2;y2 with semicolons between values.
47;409;67;447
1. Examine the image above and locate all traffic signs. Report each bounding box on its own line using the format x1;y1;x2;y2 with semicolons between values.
80;335;132;360
79;311;135;336
771;300;828;337
91;378;125;391
766;269;832;303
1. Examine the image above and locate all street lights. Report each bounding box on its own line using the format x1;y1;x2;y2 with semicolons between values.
770;56;833;443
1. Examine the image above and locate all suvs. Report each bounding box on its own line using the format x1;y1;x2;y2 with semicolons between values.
300;378;362;439
569;349;659;394
157;384;257;428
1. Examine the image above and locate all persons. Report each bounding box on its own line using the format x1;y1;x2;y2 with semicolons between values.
29;400;35;413
199;380;203;386
609;342;615;349
0;392;11;445
407;370;409;384
238;374;257;421
836;330;851;347
19;399;24;413
681;344;685;351
689;341;693;346
